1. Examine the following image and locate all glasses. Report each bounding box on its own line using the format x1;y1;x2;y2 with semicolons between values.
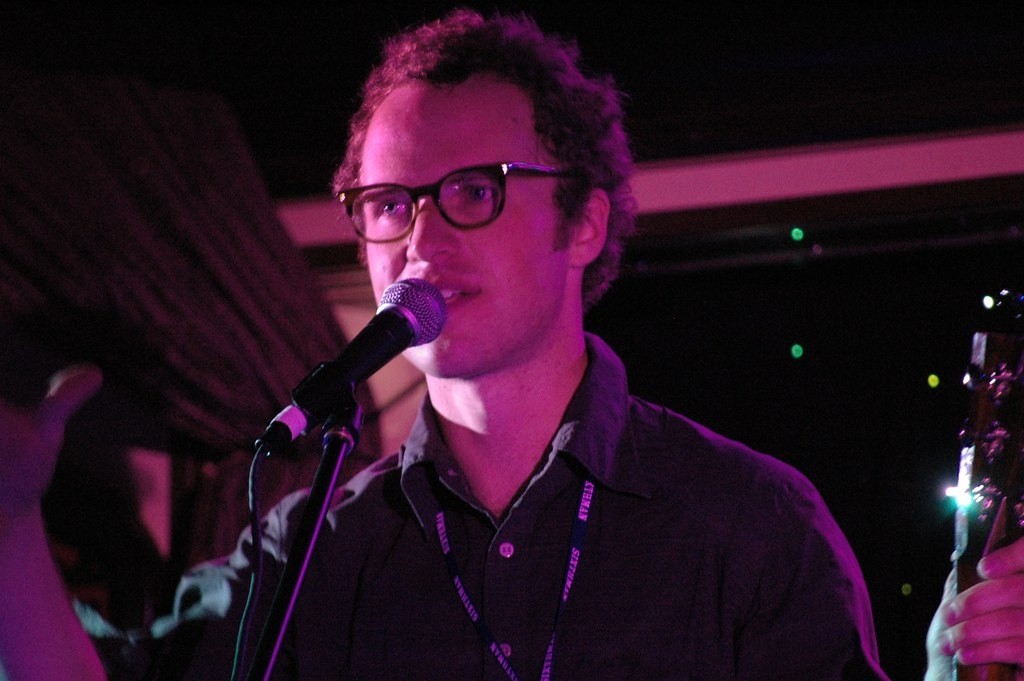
335;160;571;245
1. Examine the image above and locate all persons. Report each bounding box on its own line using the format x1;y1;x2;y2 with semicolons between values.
0;10;1024;681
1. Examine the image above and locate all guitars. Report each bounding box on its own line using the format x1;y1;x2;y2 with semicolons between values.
956;288;1024;681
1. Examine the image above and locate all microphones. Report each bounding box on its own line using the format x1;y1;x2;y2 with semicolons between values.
255;277;448;457
